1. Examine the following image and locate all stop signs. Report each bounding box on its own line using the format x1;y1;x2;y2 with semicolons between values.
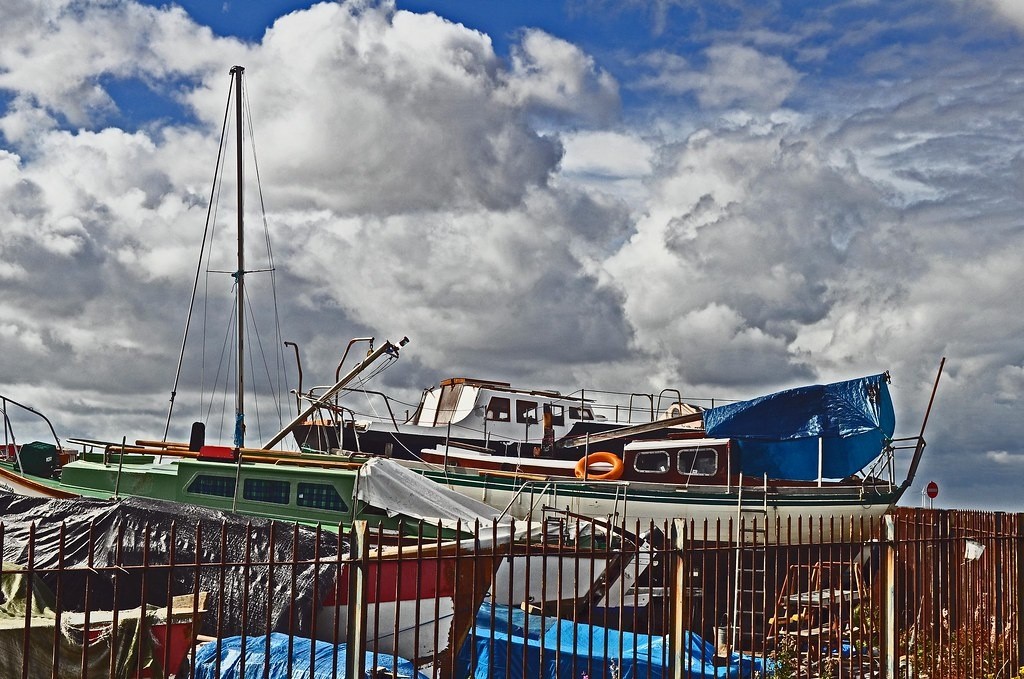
926;481;939;499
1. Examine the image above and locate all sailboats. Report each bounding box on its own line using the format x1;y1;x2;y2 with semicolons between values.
1;63;704;629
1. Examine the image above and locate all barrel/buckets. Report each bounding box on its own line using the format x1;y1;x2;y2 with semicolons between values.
711;613;740;658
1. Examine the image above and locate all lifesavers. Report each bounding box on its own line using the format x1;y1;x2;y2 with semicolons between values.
574;451;625;483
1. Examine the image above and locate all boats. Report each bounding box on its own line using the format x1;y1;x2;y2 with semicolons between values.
1;495;516;679
420;355;947;515
282;332;747;460
